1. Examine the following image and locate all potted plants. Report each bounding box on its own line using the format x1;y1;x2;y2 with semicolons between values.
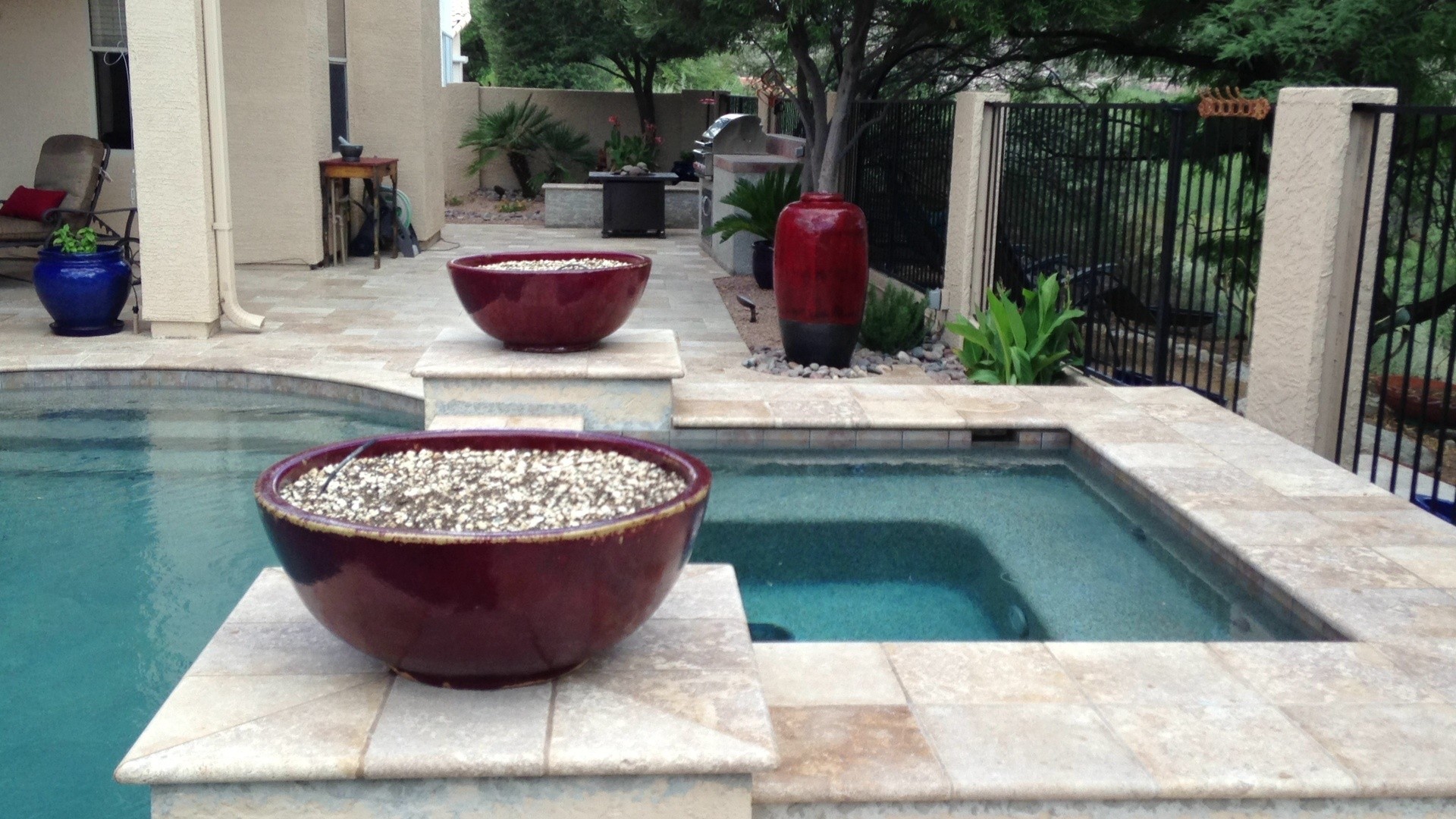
32;224;132;338
700;162;805;289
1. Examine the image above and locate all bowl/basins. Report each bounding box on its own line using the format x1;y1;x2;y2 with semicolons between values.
447;251;651;348
255;428;717;686
339;145;363;161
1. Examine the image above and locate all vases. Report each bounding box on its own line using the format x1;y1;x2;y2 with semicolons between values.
773;193;867;368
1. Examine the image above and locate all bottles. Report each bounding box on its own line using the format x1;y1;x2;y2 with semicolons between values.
774;192;869;369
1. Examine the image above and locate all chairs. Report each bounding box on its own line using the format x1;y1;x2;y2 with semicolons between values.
993;222;1217;367
0;133;142;285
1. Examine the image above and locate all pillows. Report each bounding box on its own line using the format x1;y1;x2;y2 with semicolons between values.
0;185;67;223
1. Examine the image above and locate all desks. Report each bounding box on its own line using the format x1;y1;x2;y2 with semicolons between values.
588;171;679;240
318;157;399;269
